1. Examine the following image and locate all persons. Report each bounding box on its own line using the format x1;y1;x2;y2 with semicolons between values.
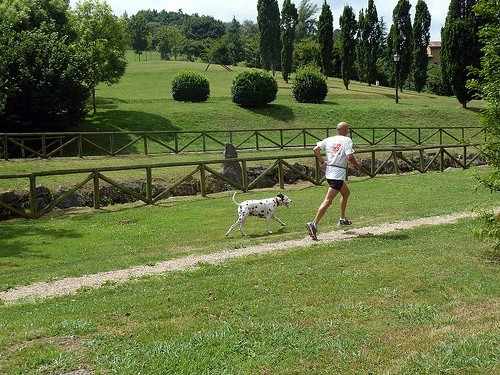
307;121;364;240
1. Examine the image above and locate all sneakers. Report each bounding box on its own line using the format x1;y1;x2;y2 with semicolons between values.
337;217;352;227
306;222;317;240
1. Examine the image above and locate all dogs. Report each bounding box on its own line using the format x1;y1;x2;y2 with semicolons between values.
225;190;292;236
